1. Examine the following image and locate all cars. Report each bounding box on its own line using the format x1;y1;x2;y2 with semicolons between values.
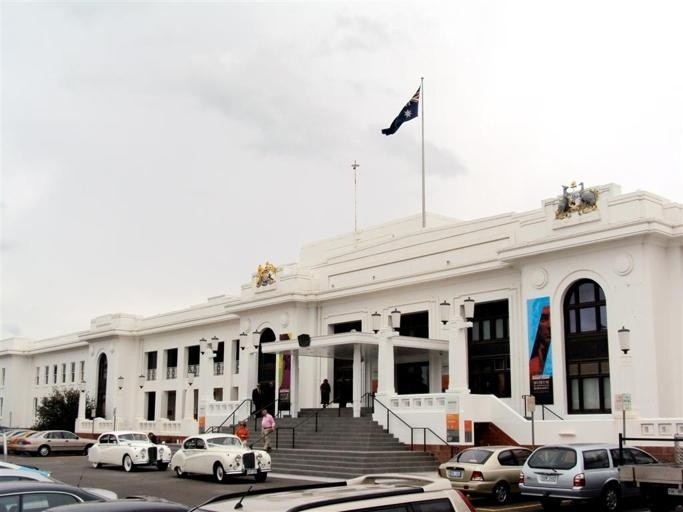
169;432;272;485
0;428;97;459
436;443;546;507
85;429;172;473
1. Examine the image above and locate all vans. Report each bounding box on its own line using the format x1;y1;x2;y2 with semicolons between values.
516;439;663;512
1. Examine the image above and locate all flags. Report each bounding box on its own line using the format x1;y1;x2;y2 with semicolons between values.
382;85;420;136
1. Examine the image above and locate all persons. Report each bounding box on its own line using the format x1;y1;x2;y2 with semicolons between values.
235;422;249;447
253;383;264;418
530;308;550;376
320;379;330;408
261;408;276;452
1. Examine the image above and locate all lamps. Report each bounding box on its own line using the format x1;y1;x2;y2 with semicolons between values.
210;336;219;353
440;300;451;325
239;332;247;351
617;326;632;357
390;307;401;331
138;374;145;389
371;311;381;334
252;329;261;349
79;379;86;393
187;370;194;386
199;337;207;355
117;376;124;390
464;296;476;322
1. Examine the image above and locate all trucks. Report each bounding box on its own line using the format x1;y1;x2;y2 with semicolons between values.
615;462;682;512
